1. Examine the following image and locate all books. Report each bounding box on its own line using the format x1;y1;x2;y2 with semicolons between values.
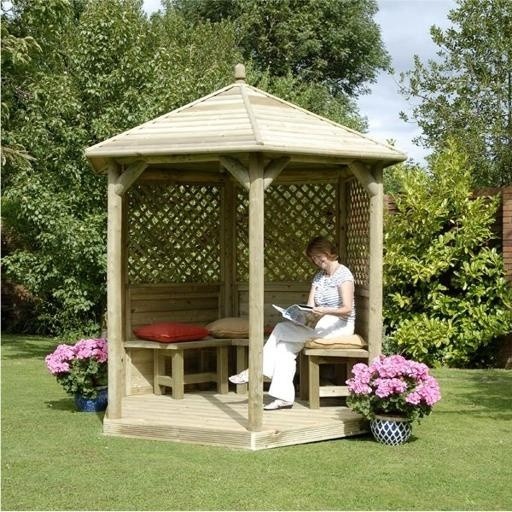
270;303;325;331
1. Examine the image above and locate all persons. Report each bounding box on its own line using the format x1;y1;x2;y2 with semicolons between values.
227;235;357;411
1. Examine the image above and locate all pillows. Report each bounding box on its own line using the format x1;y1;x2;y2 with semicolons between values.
132;315;251;344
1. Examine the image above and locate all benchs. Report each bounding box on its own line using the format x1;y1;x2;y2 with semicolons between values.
123;333;370;409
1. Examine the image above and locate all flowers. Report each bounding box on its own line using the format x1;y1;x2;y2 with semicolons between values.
343;353;443;426
43;337;108;401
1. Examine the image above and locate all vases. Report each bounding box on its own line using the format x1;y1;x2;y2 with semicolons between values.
71;388;107;413
368;410;414;447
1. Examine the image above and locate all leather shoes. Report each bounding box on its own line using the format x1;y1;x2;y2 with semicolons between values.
229;367;248;385
264;399;293;410
